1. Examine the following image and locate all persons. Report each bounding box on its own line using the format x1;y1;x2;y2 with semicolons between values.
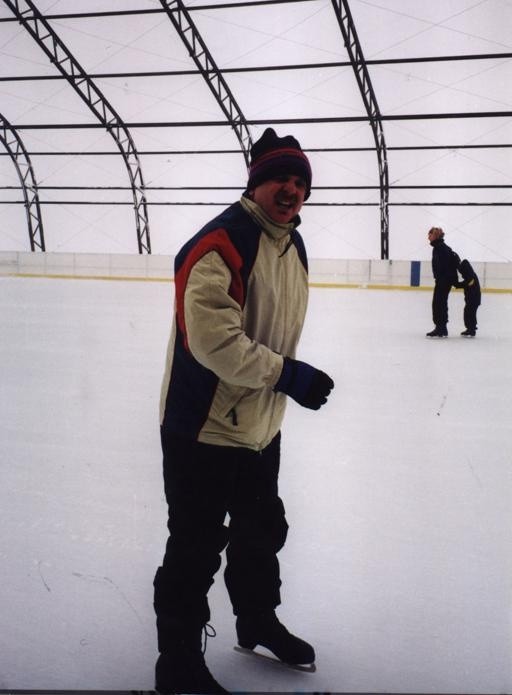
426;227;460;336
148;125;339;693
450;258;482;336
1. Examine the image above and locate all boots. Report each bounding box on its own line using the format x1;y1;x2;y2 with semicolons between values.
235;607;317;664
153;620;229;694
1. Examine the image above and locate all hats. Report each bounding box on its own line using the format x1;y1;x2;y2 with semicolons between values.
244;127;313;202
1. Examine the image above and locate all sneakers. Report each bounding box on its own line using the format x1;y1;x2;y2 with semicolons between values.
426;326;476;337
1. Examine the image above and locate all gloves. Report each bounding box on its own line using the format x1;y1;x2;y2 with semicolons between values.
272;355;335;412
